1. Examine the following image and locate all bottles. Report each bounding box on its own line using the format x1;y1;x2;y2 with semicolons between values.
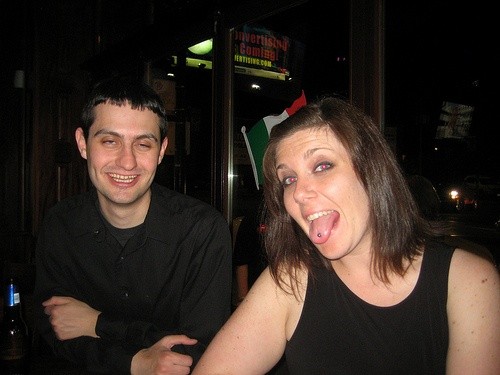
0;278;31;375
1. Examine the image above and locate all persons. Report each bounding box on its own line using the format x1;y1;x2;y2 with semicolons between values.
15;76;234;375
191;97;500;375
